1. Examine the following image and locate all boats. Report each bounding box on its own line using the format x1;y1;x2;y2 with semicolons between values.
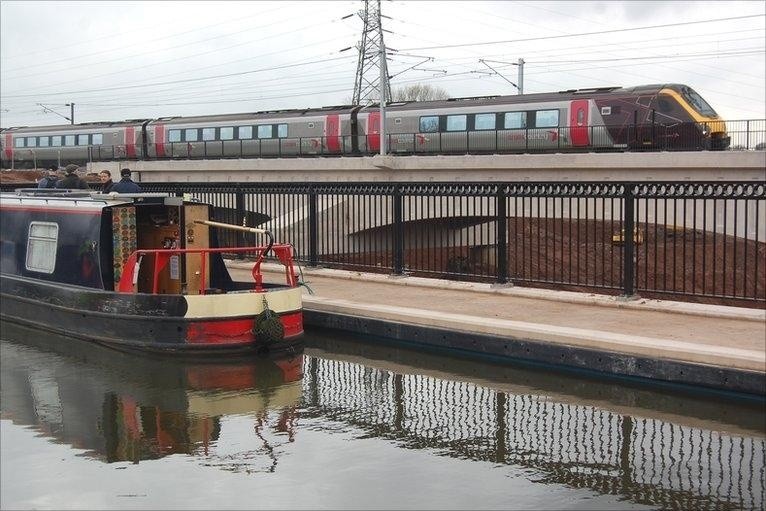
0;185;310;360
1;320;308;467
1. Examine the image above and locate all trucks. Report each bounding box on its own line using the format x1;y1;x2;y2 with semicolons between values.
612;228;644;247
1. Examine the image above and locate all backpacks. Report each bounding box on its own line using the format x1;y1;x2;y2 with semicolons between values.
45;177;60;188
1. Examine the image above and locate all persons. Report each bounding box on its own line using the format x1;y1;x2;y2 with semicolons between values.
37;165;62;188
108;168;141;193
55;164;90;189
99;170;114;194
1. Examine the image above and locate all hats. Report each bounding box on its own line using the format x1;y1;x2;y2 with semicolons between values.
121;168;131;178
48;163;59;170
65;164;79;172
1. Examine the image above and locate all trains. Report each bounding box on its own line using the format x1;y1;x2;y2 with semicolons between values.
1;79;739;169
